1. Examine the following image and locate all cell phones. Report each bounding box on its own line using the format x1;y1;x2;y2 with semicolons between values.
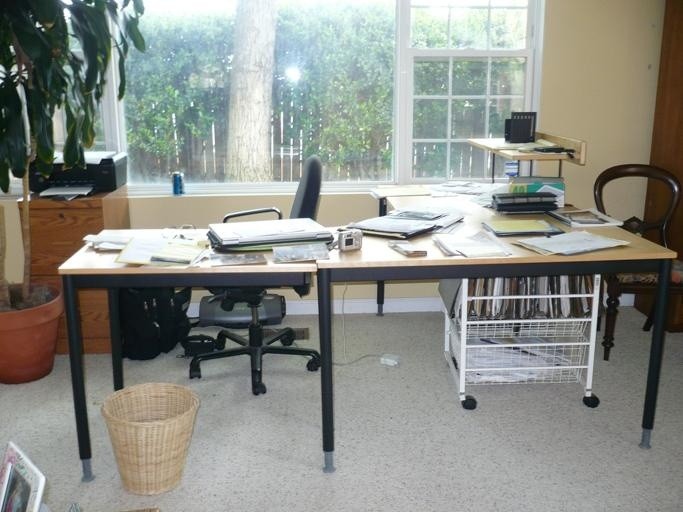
534;147;564;153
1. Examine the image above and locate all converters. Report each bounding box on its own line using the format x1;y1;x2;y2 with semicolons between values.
380;353;399;366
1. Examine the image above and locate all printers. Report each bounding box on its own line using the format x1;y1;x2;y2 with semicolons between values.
29;150;127;197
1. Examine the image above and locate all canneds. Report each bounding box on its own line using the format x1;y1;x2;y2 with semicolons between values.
172;172;185;195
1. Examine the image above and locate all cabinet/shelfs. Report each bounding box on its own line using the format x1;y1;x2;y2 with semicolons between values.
443;273;602;410
17;185;130;354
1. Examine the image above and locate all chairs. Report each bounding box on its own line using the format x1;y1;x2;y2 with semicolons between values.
595;165;681;360
190;154;321;396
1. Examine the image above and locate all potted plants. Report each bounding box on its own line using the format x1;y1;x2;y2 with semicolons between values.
0;1;146;385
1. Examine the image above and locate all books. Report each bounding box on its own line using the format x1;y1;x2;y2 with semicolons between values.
437;274;594;320
346;180;630;259
82;217;334;268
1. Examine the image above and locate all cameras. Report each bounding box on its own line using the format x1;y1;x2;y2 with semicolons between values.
338;229;362;251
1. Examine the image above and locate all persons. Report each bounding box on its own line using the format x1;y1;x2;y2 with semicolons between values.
5;479;26;512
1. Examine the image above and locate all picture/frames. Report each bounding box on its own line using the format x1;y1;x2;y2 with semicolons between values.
1;442;46;512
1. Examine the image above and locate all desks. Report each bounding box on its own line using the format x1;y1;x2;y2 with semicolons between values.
58;194;679;483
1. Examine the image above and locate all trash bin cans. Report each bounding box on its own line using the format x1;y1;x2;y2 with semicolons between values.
101;382;201;497
